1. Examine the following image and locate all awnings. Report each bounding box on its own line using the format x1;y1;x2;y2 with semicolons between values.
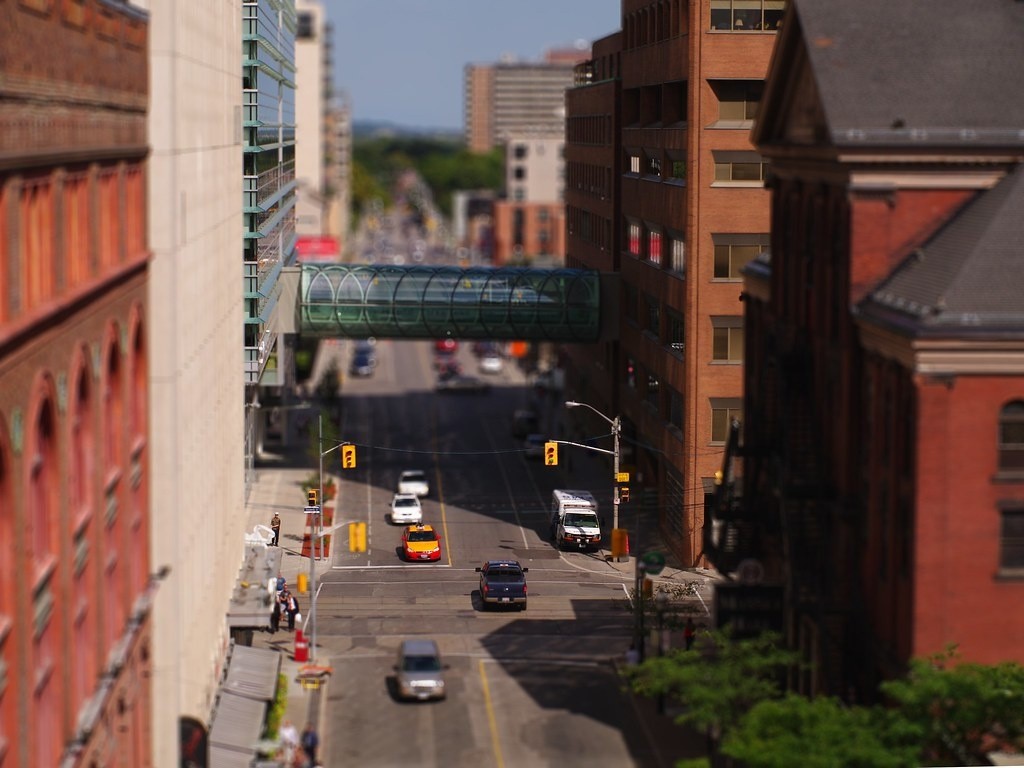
222;645;282;702
207;691;266;753
205;745;257;768
228;545;283;629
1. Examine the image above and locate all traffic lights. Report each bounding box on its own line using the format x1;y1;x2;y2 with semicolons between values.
544;442;558;465
621;487;629;503
342;445;356;468
308;490;317;506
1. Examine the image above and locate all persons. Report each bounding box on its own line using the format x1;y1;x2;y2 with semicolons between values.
271;512;281;547
274;571;299;630
299;721;319;768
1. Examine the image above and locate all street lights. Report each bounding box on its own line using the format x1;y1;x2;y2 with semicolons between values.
564;401;621;563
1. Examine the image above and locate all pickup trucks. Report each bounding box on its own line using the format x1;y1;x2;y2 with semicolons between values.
474;559;530;610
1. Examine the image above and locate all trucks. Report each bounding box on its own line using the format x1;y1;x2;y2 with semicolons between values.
549;489;602;552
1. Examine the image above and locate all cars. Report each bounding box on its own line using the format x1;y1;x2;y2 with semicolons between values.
397;468;430;498
390;491;424;525
346;338;506;396
522;433;550;458
511;409;540;439
391;639;452;702
400;522;444;563
